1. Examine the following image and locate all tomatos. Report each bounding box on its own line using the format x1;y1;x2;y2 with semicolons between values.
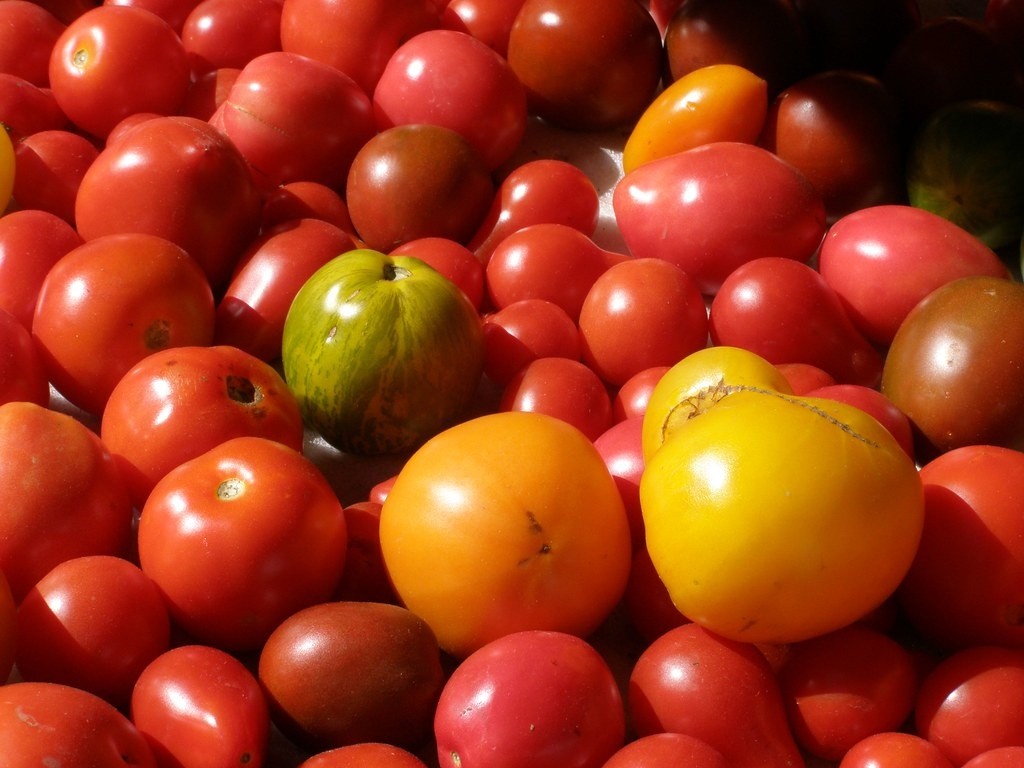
0;0;1024;768
